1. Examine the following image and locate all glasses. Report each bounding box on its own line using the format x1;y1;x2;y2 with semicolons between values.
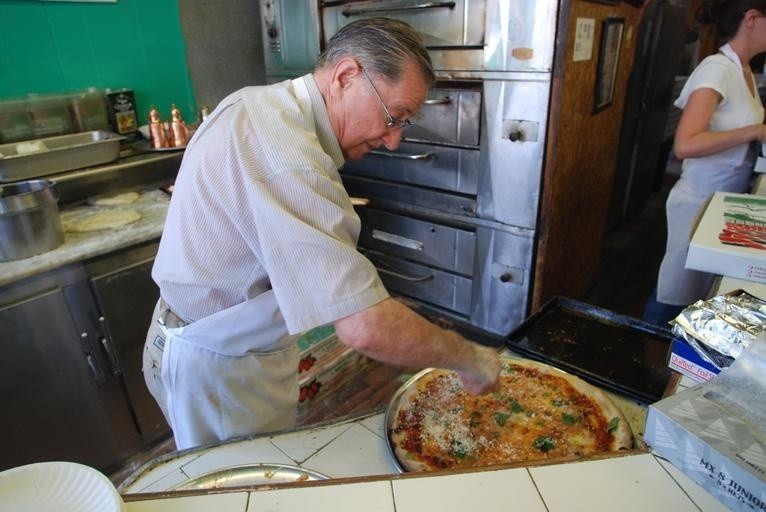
351;57;415;130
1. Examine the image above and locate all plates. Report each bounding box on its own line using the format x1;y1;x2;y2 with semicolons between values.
0;462;126;510
132;131;196;156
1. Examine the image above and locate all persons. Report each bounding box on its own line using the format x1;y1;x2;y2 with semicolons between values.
649;0;764;311
140;16;501;453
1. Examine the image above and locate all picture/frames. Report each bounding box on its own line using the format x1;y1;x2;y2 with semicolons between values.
592;16;625;117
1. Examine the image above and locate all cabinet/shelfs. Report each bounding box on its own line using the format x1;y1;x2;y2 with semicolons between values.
0;254;177;478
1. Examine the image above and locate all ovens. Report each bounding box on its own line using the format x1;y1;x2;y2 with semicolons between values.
260;1;560;336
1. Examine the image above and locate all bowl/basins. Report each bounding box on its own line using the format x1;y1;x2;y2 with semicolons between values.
137;123;172;139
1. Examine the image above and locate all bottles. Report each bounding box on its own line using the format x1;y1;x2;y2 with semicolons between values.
149;104;188;146
199;105;211;121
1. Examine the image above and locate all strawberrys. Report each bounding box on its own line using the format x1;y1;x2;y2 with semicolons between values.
298;354;323;403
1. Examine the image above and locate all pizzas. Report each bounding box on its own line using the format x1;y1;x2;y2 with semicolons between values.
392;358;634;472
